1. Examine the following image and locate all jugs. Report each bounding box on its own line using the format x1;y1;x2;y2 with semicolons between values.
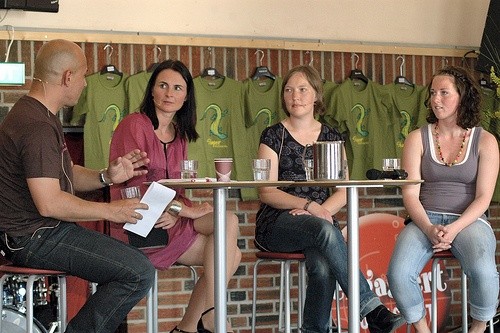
302;141;345;181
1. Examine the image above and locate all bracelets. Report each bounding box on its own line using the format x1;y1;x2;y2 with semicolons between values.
167;200;182;216
99;167;114;186
304;199;312;211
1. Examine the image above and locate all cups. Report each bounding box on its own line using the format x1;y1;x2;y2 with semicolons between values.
120;186;142;200
214;158;233;182
303;159;314;181
181;160;198;179
253;159;270;181
383;158;401;171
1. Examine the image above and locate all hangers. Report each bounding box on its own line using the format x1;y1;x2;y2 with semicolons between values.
100;45;123;77
349;53;368;85
250;49;276;82
394;56;415;89
200;47;225;80
146;46;162;74
305;52;326;83
478;78;495;91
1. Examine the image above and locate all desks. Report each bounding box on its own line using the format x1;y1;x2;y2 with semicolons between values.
142;178;424;333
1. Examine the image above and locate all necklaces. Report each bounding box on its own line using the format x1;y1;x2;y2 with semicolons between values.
435;121;468;169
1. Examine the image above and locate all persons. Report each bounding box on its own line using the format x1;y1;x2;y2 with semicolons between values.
0;39;156;333
255;65;405;333
107;59;243;333
386;66;500;333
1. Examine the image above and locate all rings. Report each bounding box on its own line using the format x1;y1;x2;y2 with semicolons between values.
135;212;139;218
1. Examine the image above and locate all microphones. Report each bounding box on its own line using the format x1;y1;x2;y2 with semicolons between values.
366;168;408;180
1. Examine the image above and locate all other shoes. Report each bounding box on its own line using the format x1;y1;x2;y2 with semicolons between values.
367;305;405;333
169;307;234;333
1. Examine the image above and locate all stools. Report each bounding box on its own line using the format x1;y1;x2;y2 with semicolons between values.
407;249;494;333
145;261;198;333
251;251;341;333
0;265;68;333
